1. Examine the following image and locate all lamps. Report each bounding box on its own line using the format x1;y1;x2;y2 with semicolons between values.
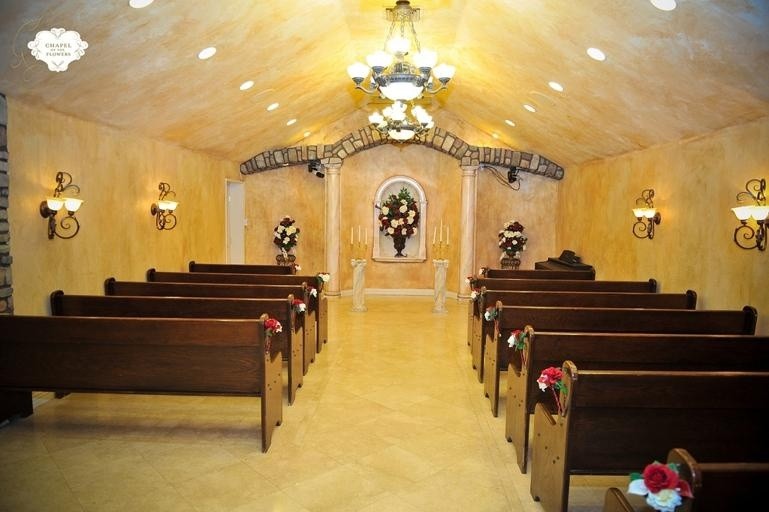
733;176;769;253
150;180;179;231
369;97;435;142
38;173;84;240
631;185;661;241
350;0;454;103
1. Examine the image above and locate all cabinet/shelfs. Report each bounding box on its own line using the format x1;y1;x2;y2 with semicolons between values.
1;265;327;454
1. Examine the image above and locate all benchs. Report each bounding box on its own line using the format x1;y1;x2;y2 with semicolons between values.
467;266;769;512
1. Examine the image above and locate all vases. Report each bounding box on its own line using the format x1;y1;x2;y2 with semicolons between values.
506;249;517;259
280;247;291;254
392;234;406;257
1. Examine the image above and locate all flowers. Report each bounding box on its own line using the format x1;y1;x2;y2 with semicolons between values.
275;214;299;252
373;184;423;241
497;216;528;258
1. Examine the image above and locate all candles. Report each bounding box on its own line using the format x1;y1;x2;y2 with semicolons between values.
433;226;437;243
446;226;449;245
440;220;442;241
358;225;361;242
365;228;367;245
350;227;354;245
